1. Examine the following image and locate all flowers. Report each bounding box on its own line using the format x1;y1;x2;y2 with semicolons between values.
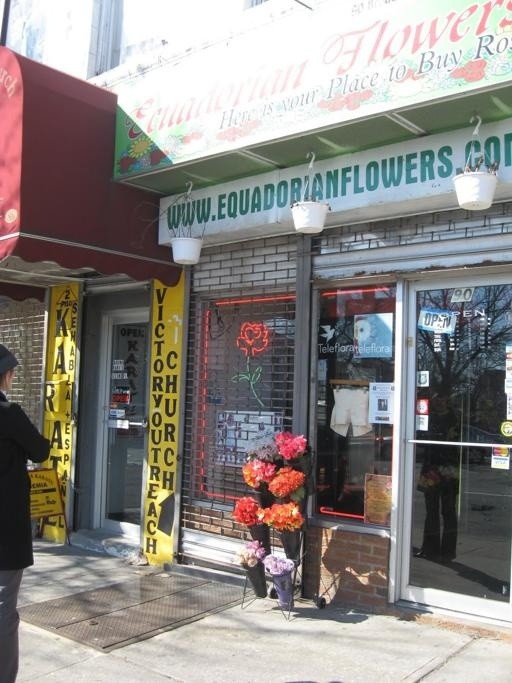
231;321;271;407
230;428;309;577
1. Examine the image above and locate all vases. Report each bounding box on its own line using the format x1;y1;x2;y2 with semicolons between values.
243;563;295;609
169;237;204;266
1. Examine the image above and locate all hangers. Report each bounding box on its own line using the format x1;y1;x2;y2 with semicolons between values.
328;368;372;391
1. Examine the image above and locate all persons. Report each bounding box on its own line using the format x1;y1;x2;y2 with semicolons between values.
0;344;50;683
417;446;459;558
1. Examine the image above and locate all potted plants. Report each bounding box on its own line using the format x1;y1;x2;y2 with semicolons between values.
290;193;332;234
453;153;500;211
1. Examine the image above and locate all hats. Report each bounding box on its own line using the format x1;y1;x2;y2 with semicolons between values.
0;344;18;374
